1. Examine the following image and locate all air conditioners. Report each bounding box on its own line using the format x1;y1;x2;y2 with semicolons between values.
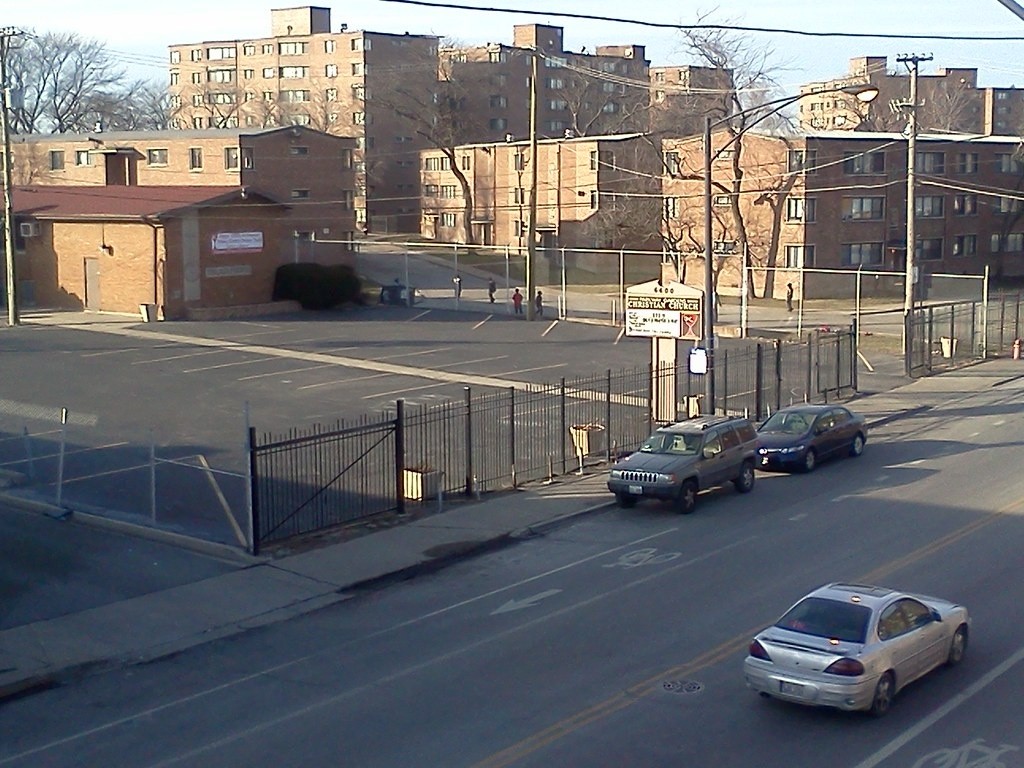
20;221;42;238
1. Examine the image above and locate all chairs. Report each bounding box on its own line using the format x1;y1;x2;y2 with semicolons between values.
671;435;687;450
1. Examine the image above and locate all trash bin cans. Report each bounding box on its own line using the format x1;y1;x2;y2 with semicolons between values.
381;285;416;306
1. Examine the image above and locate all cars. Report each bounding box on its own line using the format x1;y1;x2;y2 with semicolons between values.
755;404;867;473
741;581;971;720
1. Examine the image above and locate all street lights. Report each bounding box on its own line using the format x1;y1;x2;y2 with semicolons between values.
705;84;879;415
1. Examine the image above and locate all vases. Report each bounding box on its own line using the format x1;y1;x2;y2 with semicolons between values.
941;337;958;358
684;394;705;415
140;301;160;324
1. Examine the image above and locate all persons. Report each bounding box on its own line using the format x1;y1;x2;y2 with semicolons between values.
453;275;462;297
512;289;523;314
488;278;496;303
787;283;794;312
536;291;542;316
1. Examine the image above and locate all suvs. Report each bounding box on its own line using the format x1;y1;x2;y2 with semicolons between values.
607;415;764;516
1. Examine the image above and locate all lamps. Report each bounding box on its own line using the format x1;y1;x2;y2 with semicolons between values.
100;243;114;259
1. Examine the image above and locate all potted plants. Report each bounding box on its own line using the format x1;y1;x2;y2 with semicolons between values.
404;459;443;503
569;414;607;459
